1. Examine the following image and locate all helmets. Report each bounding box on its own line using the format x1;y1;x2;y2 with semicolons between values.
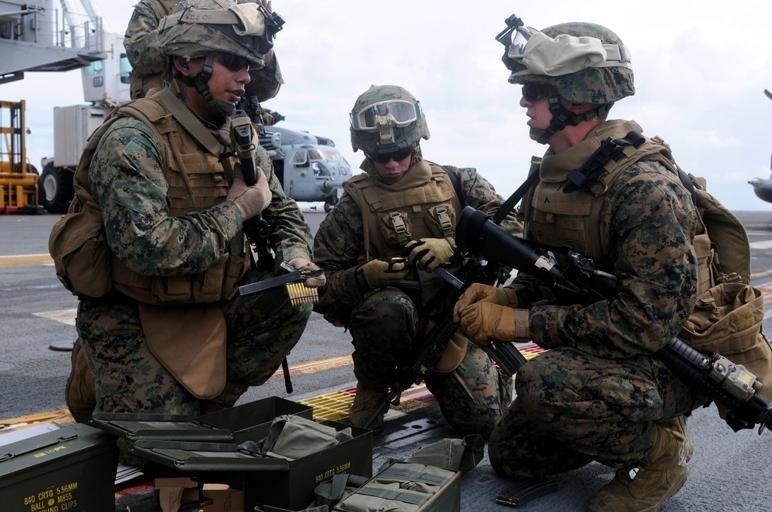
153;0;285;71
350;85;429;152
496;14;634;104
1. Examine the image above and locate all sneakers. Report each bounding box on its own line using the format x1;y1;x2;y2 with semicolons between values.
351;383;385;435
65;338;96;422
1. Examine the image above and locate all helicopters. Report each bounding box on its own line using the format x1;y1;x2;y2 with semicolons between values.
257;125;354;211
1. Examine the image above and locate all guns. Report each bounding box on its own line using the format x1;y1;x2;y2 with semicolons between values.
344;264;526;434
514;239;618;283
226;107;297;395
456;205;772;431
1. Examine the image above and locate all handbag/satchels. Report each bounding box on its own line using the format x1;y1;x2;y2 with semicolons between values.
684;280;772;408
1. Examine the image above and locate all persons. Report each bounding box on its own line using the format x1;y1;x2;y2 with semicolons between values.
308;80;520;443
61;1;325;469
117;1;291;116
452;15;753;512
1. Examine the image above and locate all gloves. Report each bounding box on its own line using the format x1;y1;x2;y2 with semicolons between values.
290;257;327;287
362;237;456;289
228;162;273;220
453;283;530;342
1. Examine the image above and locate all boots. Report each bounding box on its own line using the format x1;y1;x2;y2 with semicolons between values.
585;428;687;512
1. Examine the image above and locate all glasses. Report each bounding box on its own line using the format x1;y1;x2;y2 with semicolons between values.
522;84;552;100
354;99;418;131
217;52;246;71
509;25;542;59
370;147;410;162
